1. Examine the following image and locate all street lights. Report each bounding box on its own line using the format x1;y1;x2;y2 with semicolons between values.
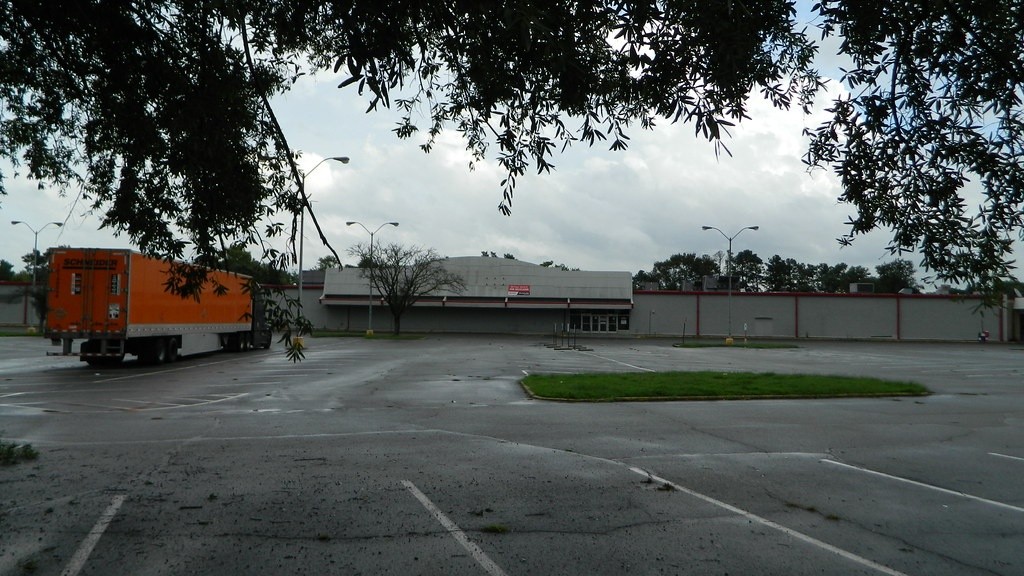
346;221;399;336
259;155;350;335
11;220;63;328
702;225;760;344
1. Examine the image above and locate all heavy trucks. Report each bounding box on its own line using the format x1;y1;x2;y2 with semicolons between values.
44;246;272;368
